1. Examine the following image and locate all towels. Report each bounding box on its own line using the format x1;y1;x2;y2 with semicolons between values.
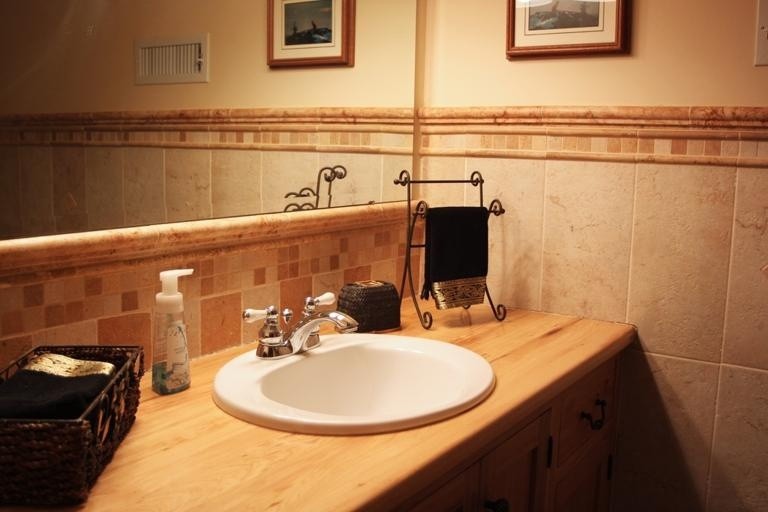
420;207;488;310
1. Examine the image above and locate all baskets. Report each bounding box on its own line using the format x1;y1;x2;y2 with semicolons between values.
0;344;143;508
337;280;400;333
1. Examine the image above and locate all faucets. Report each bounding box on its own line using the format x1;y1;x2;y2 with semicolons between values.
285;310;360;354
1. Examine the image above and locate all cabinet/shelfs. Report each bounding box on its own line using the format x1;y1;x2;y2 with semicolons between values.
391;352;624;512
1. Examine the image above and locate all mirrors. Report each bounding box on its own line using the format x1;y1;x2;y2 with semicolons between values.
1;1;428;277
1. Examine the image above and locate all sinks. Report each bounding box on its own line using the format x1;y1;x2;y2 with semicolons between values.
212;334;495;436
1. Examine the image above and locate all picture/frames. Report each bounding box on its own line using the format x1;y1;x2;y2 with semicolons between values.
266;1;356;68
505;0;625;61
